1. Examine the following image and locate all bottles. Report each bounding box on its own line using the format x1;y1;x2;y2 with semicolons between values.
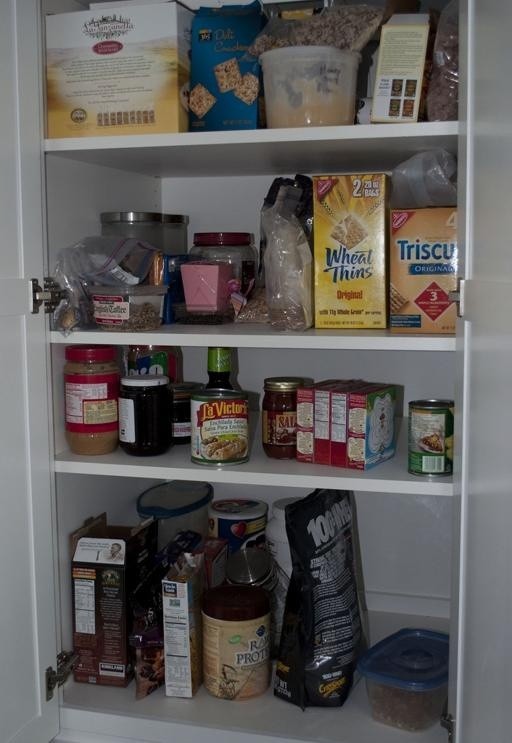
120;376;174;458
172;382;199;445
99;210;163;253
189;232;260;300
64;346;119;456
160;213;189;255
199;583;274;700
267;495;303;657
204;347;230;390
262;377;306;458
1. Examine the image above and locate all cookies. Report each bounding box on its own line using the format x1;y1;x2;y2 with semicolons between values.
189;83;217;119
331;213;368;248
214;57;241;92
390;283;408;313
233;72;259;105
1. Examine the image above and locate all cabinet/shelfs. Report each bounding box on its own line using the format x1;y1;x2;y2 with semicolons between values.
0;1;512;740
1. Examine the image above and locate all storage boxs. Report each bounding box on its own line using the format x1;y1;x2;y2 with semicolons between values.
41;0;196;137
67;510;158;688
310;171;391;332
367;13;431;124
160;550;207;699
354;625;449;734
390;206;458;336
188;2;267;133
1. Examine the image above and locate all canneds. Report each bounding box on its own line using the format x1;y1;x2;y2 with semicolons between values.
407;398;454;477
188;387;252;467
